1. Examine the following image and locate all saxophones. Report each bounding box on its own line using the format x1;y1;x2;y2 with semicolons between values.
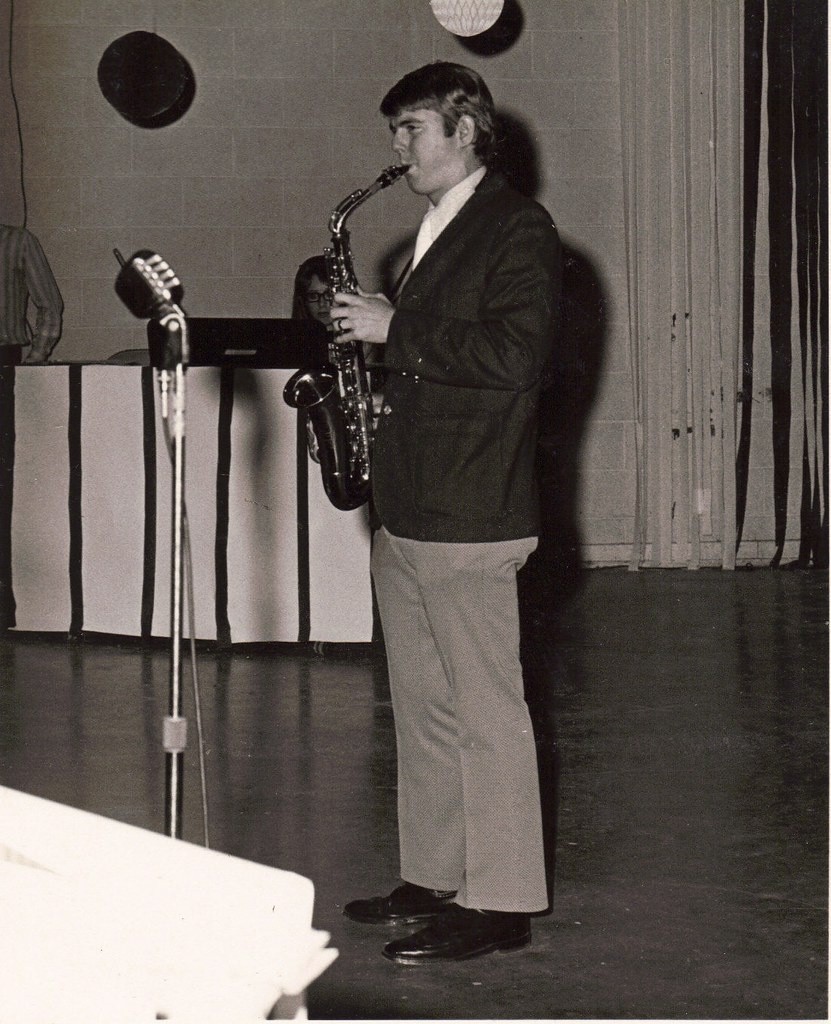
281;161;407;514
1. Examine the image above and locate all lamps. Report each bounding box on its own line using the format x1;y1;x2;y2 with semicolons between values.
430;0;505;38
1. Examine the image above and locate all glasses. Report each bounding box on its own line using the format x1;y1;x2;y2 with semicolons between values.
306;290;333;302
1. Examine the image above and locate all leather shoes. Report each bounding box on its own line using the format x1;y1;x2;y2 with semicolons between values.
344;880;458;927
381;903;531;965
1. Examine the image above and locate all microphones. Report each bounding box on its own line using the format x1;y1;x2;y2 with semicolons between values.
116;249;188;373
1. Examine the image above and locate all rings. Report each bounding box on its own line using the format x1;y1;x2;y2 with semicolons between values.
338;319;346;333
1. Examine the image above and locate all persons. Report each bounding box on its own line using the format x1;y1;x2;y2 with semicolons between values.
292;256;334;344
330;62;563;965
1;221;63;640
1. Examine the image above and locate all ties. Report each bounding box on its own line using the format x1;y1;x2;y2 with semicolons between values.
411;209;434;270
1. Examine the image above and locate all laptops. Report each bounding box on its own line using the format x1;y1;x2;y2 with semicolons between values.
184;316;329;371
